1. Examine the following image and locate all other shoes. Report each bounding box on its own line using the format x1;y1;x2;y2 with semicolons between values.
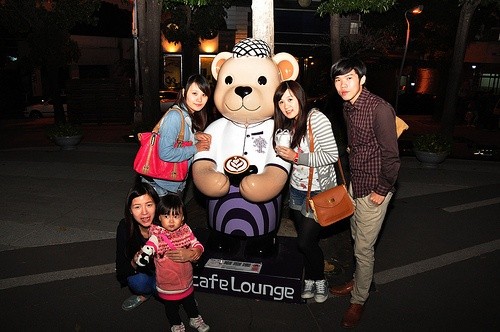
121;294;149;311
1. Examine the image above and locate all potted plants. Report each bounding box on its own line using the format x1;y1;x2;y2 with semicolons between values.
402;130;454;163
45;120;83;145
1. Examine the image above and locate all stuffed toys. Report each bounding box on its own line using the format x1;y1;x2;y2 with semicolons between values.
136;245;154;266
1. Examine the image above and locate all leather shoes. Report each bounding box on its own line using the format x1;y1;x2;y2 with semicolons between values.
343;302;363;327
330;282;354;296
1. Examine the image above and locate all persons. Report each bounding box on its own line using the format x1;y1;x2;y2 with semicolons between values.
133;194;211;332
115;180;199;310
134;76;217;223
330;56;400;327
271;80;342;303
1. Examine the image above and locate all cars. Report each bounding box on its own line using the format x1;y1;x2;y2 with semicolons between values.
133;90;180;114
23;93;68;118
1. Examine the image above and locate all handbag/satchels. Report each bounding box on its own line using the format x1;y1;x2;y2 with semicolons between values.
310;184;356;226
133;109;188;182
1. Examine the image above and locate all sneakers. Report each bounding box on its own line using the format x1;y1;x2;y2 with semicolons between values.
301;279;316;298
171;324;185;332
314;278;329;303
189;314;209;332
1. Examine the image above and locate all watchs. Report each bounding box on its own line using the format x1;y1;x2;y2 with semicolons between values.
294;152;298;164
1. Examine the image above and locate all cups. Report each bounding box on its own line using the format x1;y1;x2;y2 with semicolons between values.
277;131;289;148
